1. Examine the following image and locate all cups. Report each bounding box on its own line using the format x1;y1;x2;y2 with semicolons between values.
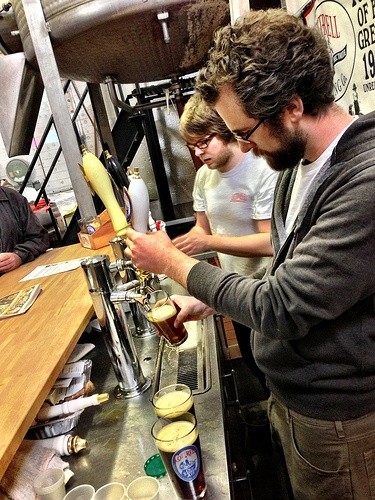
77;215;103;234
138;290;188;347
151;383;195;426
126;476;160;500
33;468;66;500
151;411;207;500
62;484;95;500
93;482;126;500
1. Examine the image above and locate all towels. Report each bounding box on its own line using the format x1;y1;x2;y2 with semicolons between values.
0;438;74;500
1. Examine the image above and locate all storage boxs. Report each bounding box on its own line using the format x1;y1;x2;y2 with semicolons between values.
78;207;126;249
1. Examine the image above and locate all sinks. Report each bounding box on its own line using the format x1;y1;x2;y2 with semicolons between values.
152;275;212;396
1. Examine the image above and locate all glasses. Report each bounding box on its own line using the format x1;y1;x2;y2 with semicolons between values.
234;117;266;144
187;131;218;150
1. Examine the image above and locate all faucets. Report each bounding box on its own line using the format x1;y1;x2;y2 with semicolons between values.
79;253;152;400
106;235;155;339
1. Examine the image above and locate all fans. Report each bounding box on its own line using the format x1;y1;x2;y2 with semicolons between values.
0;155;34;187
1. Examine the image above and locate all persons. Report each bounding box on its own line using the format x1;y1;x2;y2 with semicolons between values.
170;93;279;426
123;9;375;500
0;186;49;277
